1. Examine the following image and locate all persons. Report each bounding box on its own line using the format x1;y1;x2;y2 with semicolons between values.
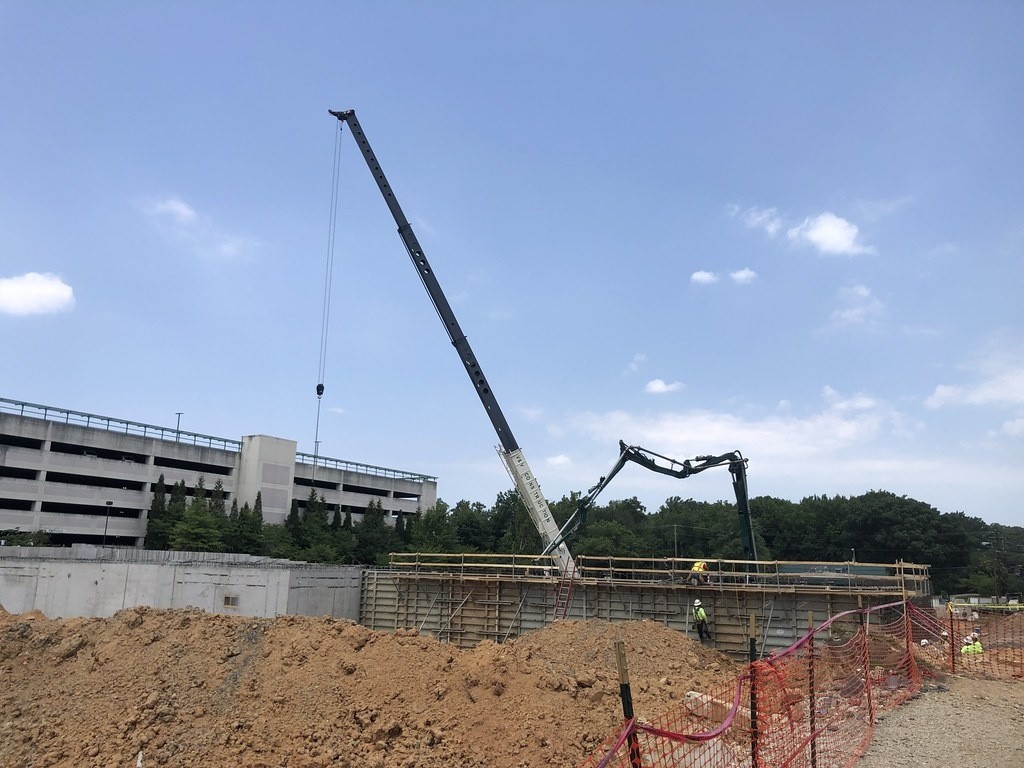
693;599;715;644
960;637;976;655
937;632;949;645
970;633;983;654
691;561;708;586
961;608;967;621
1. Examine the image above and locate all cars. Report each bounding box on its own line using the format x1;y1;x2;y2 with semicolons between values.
1006;592;1022;603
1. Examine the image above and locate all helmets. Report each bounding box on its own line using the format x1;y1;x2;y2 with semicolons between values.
694;599;701;606
970;632;979;638
941;631;949;636
964;636;973;644
921;639;929;645
